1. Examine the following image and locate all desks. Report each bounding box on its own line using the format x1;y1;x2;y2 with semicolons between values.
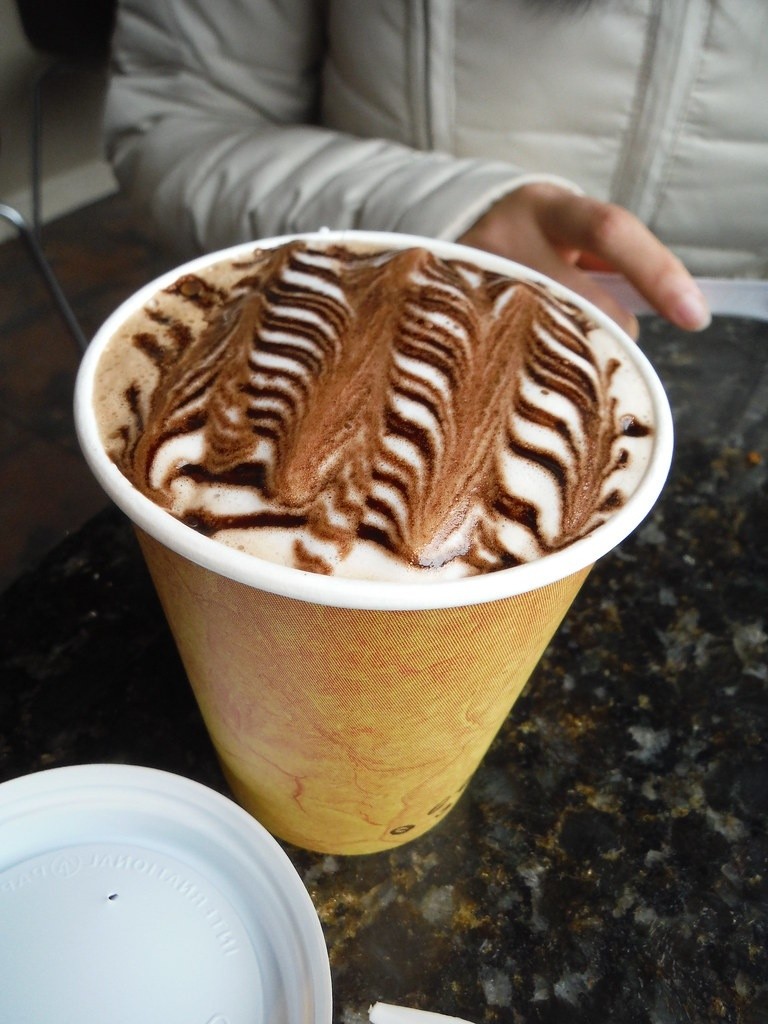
0;311;768;1024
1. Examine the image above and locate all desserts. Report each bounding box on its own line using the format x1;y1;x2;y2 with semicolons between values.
94;241;658;580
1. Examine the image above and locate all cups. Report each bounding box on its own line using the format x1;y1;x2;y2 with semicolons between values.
73;228;672;855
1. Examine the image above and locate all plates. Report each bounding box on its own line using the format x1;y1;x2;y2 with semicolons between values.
0;763;333;1024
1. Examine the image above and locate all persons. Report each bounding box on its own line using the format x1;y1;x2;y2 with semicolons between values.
103;0;768;347
